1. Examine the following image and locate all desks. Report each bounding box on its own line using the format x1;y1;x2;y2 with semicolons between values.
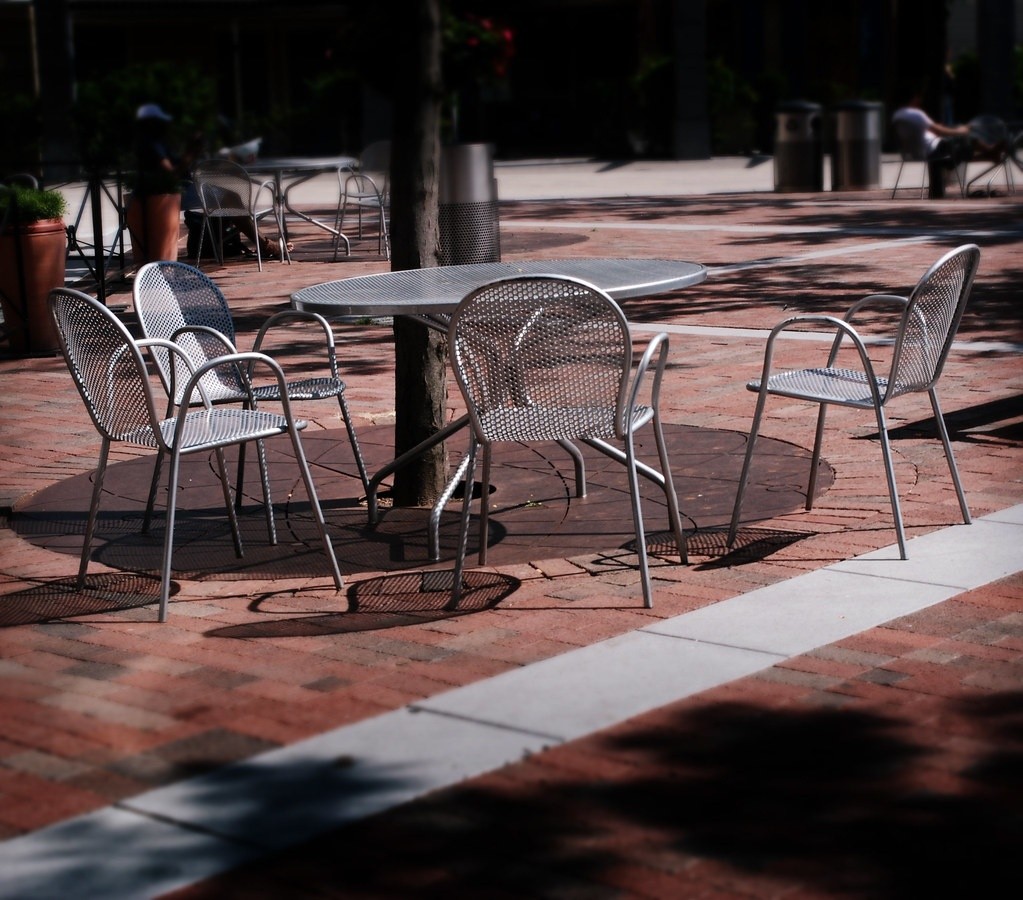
290;257;708;563
200;157;359;263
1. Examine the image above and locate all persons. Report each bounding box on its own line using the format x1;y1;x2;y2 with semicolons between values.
137;106;283;257
889;89;1009;165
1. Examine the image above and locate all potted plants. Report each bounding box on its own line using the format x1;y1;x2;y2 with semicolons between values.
0;182;67;357
111;170;191;266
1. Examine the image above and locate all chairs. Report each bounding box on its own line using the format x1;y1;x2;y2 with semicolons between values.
447;272;689;608
194;158;291;272
45;287;344;624
332;141;391;267
891;110;1023;200
726;244;981;562
132;260;369;545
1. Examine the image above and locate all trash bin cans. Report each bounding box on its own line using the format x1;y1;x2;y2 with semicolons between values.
440;143;504;264
772;101;826;193
831;99;883;191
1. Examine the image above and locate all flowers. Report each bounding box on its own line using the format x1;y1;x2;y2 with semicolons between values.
439;14;516;79
